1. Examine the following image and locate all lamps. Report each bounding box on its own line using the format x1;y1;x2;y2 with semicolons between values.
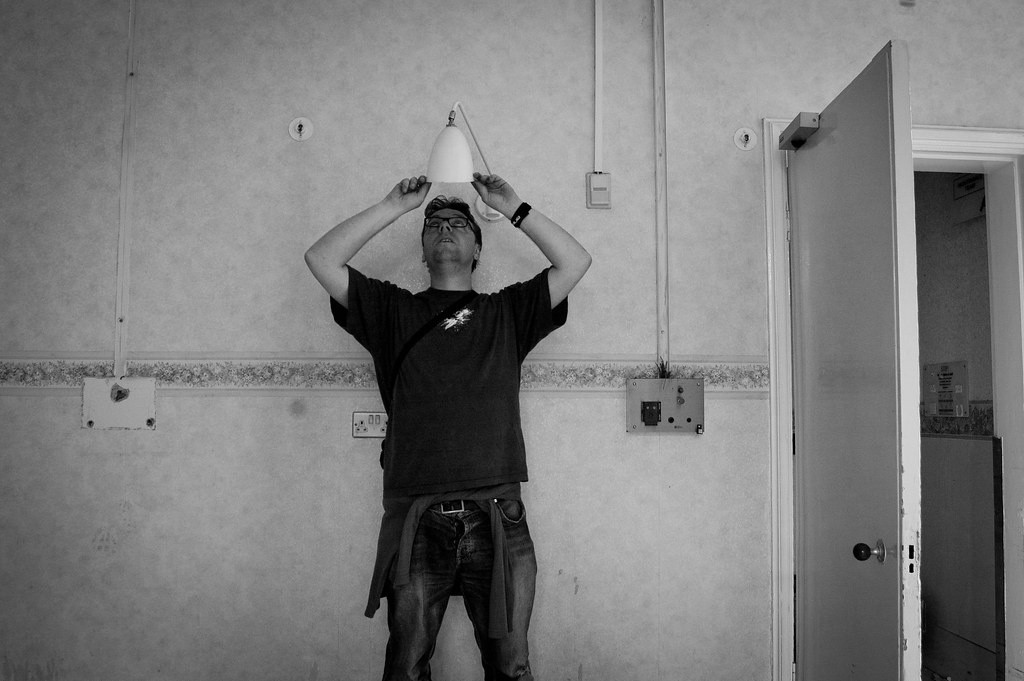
426;100;510;223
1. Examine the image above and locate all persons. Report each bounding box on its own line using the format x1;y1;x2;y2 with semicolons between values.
305;173;593;680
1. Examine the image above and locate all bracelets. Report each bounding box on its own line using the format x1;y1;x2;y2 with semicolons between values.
509;201;531;228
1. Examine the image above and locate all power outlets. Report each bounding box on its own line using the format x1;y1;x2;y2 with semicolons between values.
353;411;389;438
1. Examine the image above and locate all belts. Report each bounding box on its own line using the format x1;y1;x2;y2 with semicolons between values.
427;499;478;515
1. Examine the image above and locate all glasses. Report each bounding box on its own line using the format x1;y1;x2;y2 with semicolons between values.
421;216;475;241
